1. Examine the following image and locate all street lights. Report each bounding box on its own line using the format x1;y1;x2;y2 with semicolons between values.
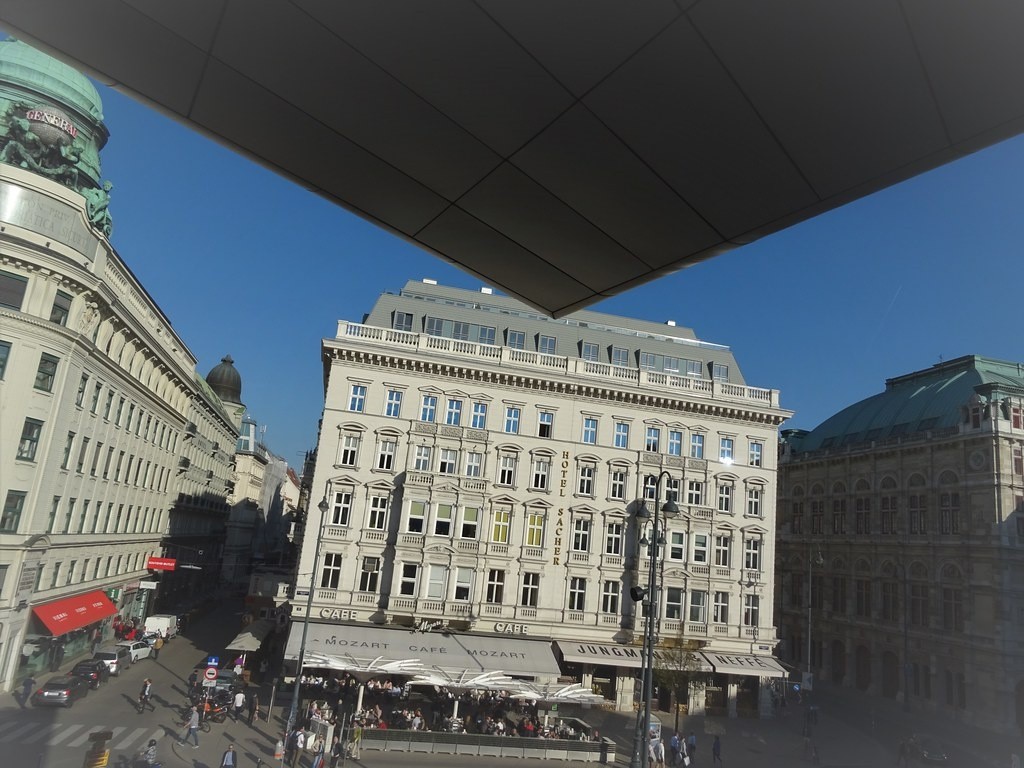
630;518;668;768
283;477;334;744
807;537;825;673
641;471;681;768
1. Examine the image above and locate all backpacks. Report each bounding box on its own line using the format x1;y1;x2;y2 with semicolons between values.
288;730;303;749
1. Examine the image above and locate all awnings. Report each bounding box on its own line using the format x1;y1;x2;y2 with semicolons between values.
554;639;788;679
33;590;119;636
281;622;562;678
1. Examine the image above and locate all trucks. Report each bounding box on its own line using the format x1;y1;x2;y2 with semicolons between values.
143;614;177;644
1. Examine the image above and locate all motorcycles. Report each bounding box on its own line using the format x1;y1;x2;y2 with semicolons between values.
182;686;233;733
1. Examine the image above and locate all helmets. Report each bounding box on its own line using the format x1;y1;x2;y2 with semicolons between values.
149;740;155;746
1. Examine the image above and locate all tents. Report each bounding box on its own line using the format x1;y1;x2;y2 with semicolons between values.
225;619;275;667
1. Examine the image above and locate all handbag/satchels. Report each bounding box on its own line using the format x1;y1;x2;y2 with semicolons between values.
254;711;258;721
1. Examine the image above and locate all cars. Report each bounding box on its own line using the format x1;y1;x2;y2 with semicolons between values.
907;733;950;766
33;675;90;708
214;668;240;694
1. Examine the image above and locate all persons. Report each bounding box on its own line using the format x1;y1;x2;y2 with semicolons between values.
140;739;157;767
299;670;449;733
286;715;363;767
647;729;724;767
90;615;163;660
136;677;156;714
230;653;270;729
176;706;200;749
219;744;237;767
20;674;37;710
465;690;602;742
51;643;65;672
186;670;198;699
780;696;790;720
895;735;910;767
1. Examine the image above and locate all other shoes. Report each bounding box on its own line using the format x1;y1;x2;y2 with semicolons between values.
152;707;156;712
137;711;144;714
192;745;199;749
178;741;185;746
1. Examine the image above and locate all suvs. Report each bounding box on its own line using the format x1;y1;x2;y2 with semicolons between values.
90;645;132;676
116;641;152;665
67;659;110;691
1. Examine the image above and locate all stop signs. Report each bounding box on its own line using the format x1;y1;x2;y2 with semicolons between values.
204;667;219;680
